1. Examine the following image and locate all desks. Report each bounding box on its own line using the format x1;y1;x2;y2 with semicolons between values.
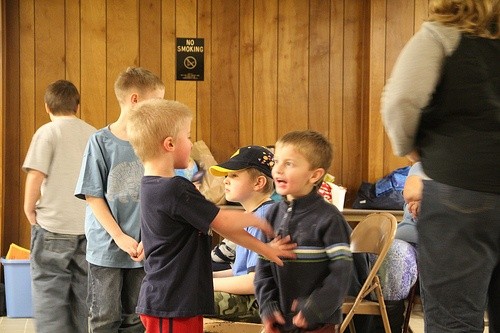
215;206;404;225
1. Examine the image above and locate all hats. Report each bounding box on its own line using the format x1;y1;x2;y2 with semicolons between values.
209;144;275;180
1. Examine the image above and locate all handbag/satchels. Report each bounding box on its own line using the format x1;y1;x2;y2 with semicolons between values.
352;180;406;211
317;180;347;214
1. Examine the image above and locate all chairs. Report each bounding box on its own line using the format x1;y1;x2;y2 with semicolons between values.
334;213;398;332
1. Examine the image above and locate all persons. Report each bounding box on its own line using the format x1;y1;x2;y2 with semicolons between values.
76;67;166;333
206;144;280;295
22;79;101;333
254;131;353;333
379;0;500;333
129;97;297;333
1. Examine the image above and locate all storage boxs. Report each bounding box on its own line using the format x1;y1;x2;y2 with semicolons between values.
0;260;35;318
